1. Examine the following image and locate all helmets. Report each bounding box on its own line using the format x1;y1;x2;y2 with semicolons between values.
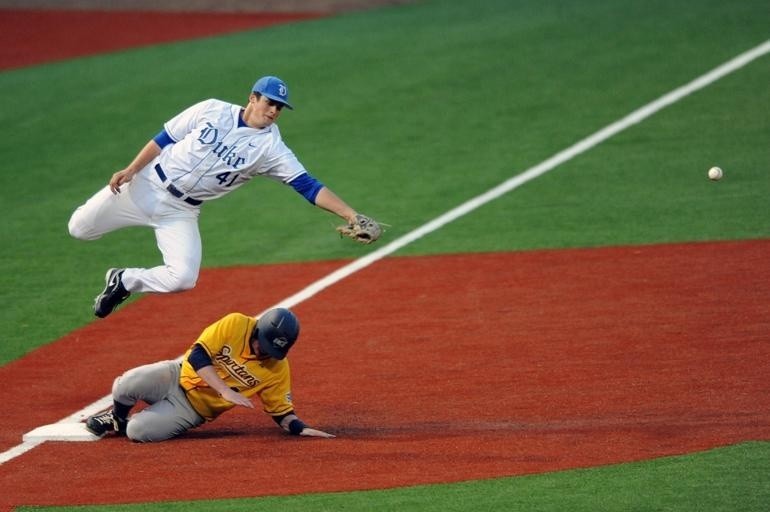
252;308;300;360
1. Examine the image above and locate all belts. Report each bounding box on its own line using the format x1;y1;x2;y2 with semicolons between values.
155;163;204;206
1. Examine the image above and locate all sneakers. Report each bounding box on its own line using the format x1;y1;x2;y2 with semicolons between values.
92;268;131;318
84;409;129;436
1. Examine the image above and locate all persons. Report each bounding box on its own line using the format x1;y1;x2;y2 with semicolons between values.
63;74;386;323
87;306;337;441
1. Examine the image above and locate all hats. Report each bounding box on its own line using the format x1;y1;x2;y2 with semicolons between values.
251;76;294;110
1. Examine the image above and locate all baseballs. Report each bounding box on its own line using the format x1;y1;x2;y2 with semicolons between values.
706;166;724;181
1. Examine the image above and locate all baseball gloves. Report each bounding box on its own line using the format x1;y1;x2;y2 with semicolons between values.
335;213;383;244
